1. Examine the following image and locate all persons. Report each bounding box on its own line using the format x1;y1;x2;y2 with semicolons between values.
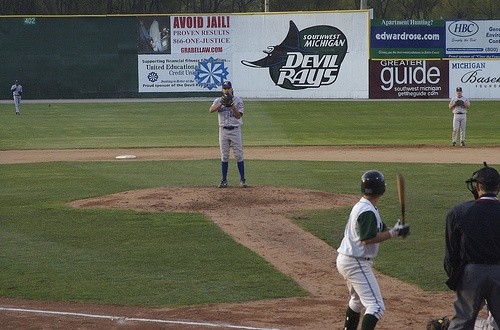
449;87;470;147
444;167;500;330
335;170;411;330
209;81;248;187
11;80;22;115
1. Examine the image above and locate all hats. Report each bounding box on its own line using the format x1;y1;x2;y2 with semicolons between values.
477;167;500;185
222;80;232;89
456;87;462;91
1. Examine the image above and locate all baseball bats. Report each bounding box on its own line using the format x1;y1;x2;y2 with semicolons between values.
397;175;407;240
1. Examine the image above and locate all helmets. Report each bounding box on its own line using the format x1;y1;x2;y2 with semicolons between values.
361;170;385;195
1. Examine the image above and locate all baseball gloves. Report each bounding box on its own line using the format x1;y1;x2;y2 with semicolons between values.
219;92;234;109
455;99;467;107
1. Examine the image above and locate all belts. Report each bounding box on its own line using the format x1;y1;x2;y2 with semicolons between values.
223;125;238;130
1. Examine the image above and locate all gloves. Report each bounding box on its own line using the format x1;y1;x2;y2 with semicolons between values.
388;218;410;240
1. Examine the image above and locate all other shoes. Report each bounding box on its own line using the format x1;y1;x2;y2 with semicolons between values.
460;142;464;147
218;180;227;188
452;142;457;147
240;181;247;187
